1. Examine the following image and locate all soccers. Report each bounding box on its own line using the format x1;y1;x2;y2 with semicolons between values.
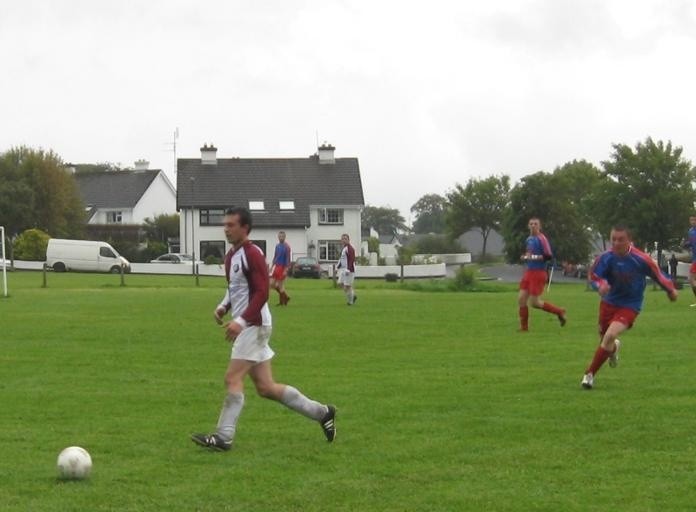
57;445;92;481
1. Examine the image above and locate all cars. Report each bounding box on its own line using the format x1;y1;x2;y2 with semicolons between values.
562;264;588;278
151;253;204;264
292;256;320;278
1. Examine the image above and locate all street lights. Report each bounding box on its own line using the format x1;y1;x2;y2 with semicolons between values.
190;176;197;274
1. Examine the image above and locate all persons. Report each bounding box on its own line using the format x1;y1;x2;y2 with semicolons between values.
660;254;677;280
580;226;678;391
269;231;291;305
191;208;338;453
679;214;696;306
519;217;567;333
337;234;357;305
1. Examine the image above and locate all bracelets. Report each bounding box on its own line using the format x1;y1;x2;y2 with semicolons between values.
346;269;350;273
233;316;248;328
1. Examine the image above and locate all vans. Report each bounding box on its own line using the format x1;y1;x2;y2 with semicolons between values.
45;239;131;273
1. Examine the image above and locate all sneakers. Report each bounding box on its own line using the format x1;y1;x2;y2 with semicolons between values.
277;297;290;305
609;339;621;368
347;295;357;305
560;307;567;326
579;371;594;388
189;432;232;451
320;405;336;441
